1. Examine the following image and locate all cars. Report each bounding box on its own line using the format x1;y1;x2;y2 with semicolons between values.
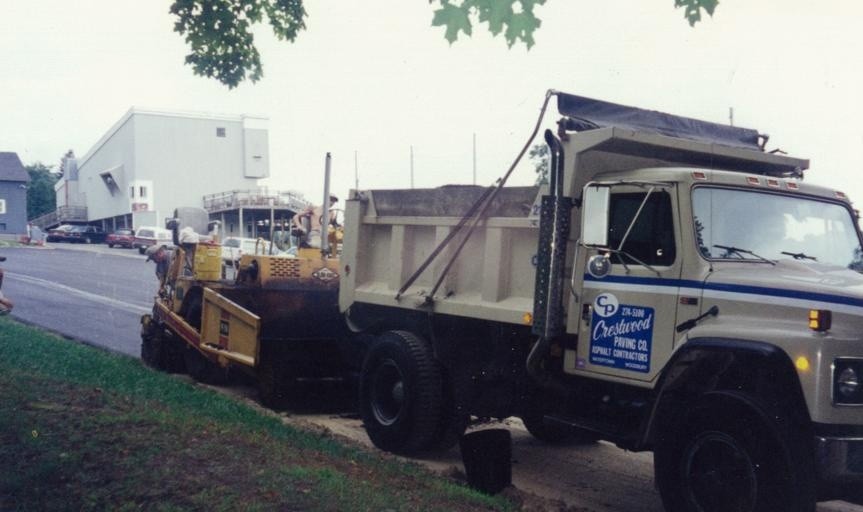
328;247;343;258
276;246;296;258
47;224;75;243
64;226;109;245
104;228;136;249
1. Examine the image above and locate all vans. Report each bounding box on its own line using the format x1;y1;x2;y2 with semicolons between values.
221;235;279;266
134;227;175;255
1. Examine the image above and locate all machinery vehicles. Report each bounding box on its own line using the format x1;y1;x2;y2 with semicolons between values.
139;152;347;407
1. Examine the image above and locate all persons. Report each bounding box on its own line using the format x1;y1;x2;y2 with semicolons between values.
144;242;193;297
291;194;344;259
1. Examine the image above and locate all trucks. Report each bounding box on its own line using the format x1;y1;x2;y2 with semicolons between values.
339;89;862;512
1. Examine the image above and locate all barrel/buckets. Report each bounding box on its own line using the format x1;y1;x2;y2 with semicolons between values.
192;241;222;281
460;428;513;496
